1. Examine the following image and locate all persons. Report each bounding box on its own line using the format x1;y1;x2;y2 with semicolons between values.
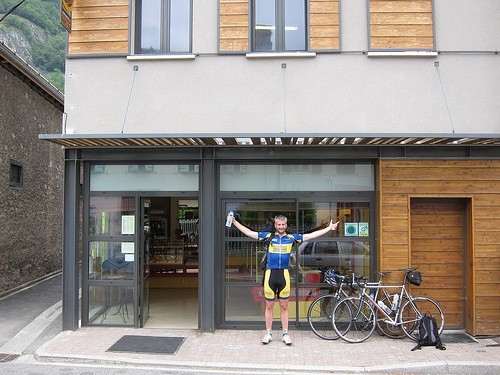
233;215;340;343
235;213;330;232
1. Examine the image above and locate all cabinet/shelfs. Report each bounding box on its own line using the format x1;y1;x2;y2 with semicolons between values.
144;238;185;275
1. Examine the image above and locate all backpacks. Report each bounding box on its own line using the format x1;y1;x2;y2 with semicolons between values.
260;233;275;268
411;311;446;351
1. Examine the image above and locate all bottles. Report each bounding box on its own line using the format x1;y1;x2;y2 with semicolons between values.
226;209;234;227
366;293;374;309
391;293;399;310
378;300;392;315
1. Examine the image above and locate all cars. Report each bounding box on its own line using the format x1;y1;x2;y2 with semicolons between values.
289;236;370;274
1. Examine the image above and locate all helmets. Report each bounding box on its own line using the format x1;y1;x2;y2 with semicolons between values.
324;269;337;287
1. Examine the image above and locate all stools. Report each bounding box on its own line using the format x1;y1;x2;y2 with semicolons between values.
100;272;130;324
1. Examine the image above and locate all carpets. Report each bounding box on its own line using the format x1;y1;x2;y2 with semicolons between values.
105;334;187;355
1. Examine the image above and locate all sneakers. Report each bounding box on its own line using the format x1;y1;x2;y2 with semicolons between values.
262;334;272;344
282;335;292;344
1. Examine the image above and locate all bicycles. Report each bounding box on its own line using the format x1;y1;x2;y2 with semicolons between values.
332;266;444;343
308;271;418;340
